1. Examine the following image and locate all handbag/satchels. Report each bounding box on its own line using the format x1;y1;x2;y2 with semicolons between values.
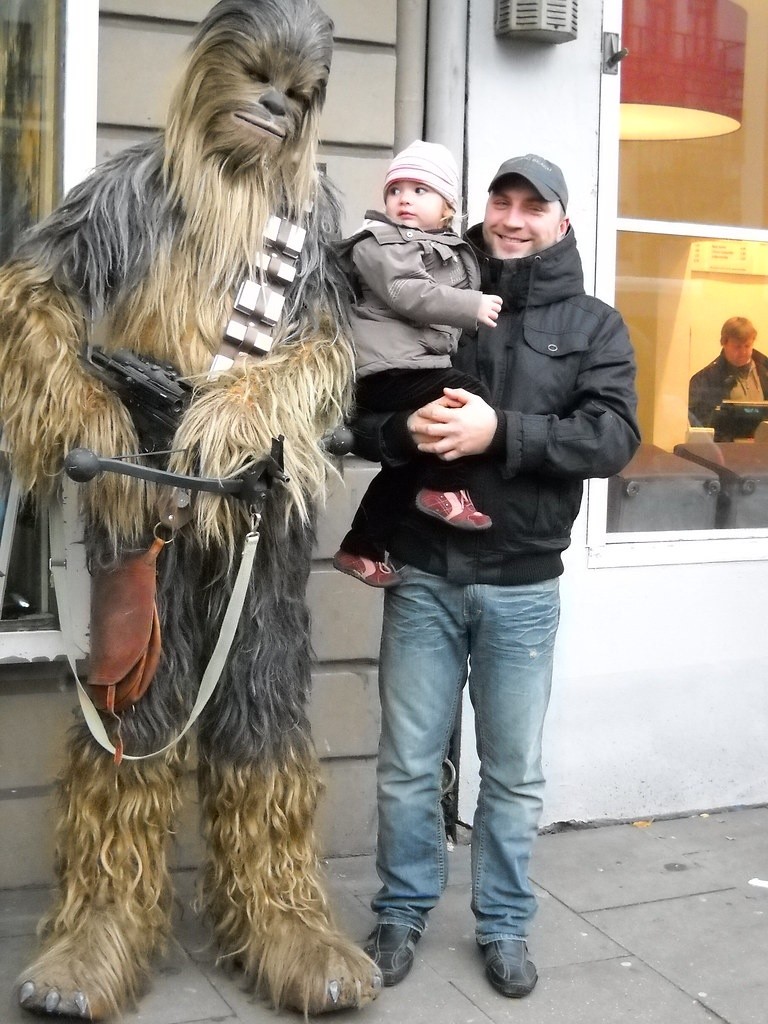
85;537;170;765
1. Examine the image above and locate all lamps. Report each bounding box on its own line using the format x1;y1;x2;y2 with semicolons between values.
619;0;750;140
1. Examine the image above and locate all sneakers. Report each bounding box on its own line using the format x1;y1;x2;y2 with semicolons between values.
332;551;402;588
484;940;538;998
364;923;421;986
416;491;491;529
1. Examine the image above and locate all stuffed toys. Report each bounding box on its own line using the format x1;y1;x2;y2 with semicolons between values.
0;1;383;1021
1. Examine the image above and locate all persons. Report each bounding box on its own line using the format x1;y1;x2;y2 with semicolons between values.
687;317;768;427
363;152;642;998
329;141;503;585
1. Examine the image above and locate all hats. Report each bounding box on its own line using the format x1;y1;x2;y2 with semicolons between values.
488;154;568;213
383;140;457;213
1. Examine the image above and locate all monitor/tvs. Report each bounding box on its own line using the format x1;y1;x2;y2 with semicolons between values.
716;400;768;440
